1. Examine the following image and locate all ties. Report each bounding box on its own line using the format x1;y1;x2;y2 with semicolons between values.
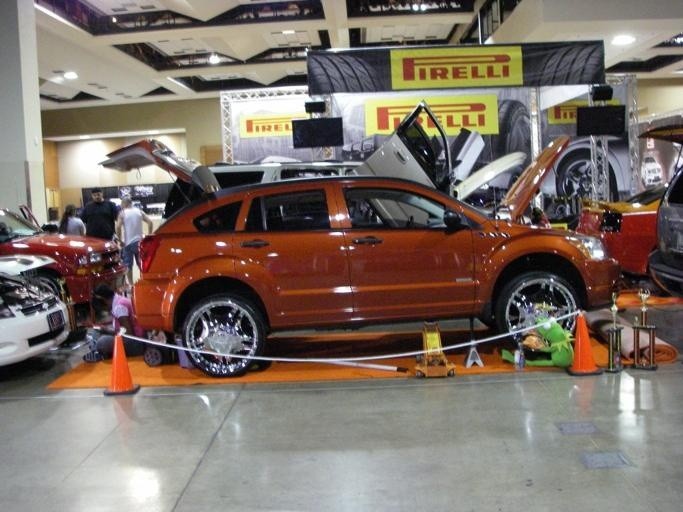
292;117;344;149
577;105;626;137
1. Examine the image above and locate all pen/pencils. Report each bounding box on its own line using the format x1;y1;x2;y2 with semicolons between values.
164;162;364;221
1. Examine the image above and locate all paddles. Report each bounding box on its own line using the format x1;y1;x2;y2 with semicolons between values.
48;308;678;387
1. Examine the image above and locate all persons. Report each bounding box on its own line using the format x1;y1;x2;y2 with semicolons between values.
78;187;120;242
82;279;145;362
116;197;152;285
161;179;192;218
46;206;59;228
58;204;86;236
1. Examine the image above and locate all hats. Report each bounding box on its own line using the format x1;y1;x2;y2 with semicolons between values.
97;133;621;378
0;254;71;367
635;122;682;300
574;181;669;277
97;98;527;227
0;204;128;305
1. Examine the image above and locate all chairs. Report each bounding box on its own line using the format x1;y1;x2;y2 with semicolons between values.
81;350;107;363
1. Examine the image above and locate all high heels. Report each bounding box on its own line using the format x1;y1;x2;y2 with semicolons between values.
565;314;602;376
103;336;139;396
